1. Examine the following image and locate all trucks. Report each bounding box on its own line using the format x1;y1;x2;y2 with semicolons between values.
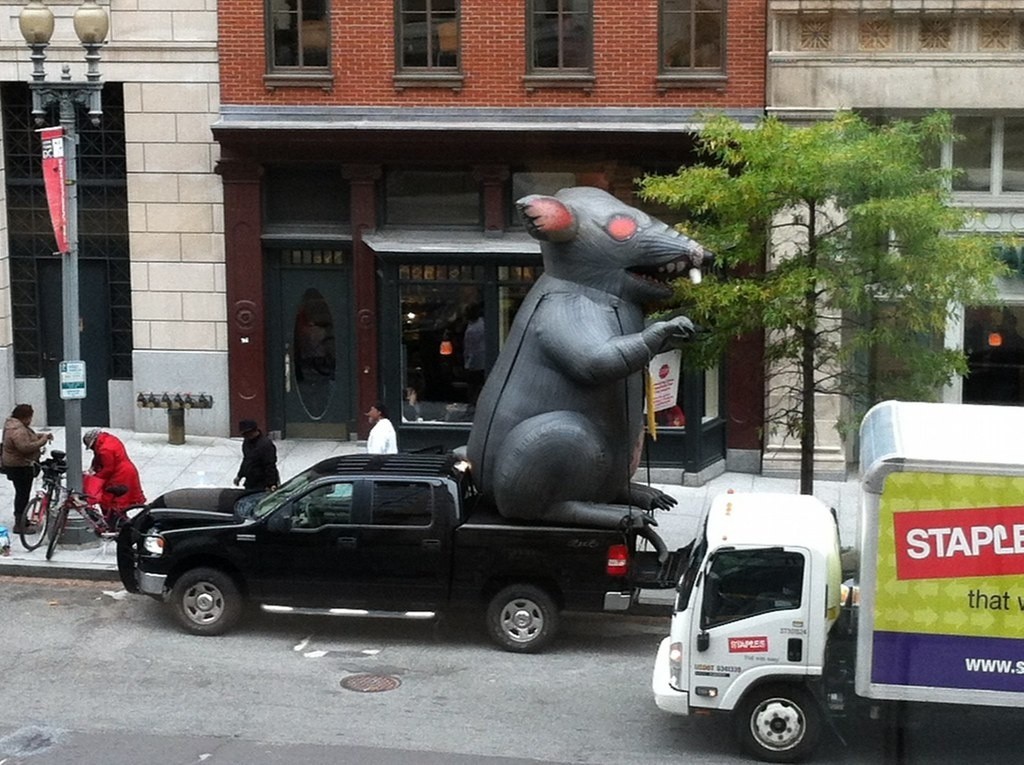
656;401;1024;764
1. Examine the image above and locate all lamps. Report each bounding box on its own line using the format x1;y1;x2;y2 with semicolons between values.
439;330;452;355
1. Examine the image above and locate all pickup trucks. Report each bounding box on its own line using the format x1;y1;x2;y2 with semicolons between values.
114;451;637;655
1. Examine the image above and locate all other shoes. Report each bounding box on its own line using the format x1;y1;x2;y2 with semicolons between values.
13;514;34;532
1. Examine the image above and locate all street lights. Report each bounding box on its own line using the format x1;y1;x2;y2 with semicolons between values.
14;0;113;546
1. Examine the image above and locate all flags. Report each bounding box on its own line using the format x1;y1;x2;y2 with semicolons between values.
39;125;74;255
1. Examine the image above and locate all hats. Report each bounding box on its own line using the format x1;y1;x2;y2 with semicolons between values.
238;419;257;434
83;429;100;448
11;403;33;418
372;401;390;417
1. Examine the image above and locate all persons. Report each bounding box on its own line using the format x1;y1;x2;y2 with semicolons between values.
84;428;146;543
233;418;280;491
461;299;485;388
2;402;53;534
364;400;399;455
405;388;422;421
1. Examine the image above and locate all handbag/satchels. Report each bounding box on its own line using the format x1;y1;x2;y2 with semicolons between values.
0;443;5;473
82;472;107;504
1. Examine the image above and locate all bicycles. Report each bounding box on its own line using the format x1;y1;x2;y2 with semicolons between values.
15;452;67;549
47;479;151;560
